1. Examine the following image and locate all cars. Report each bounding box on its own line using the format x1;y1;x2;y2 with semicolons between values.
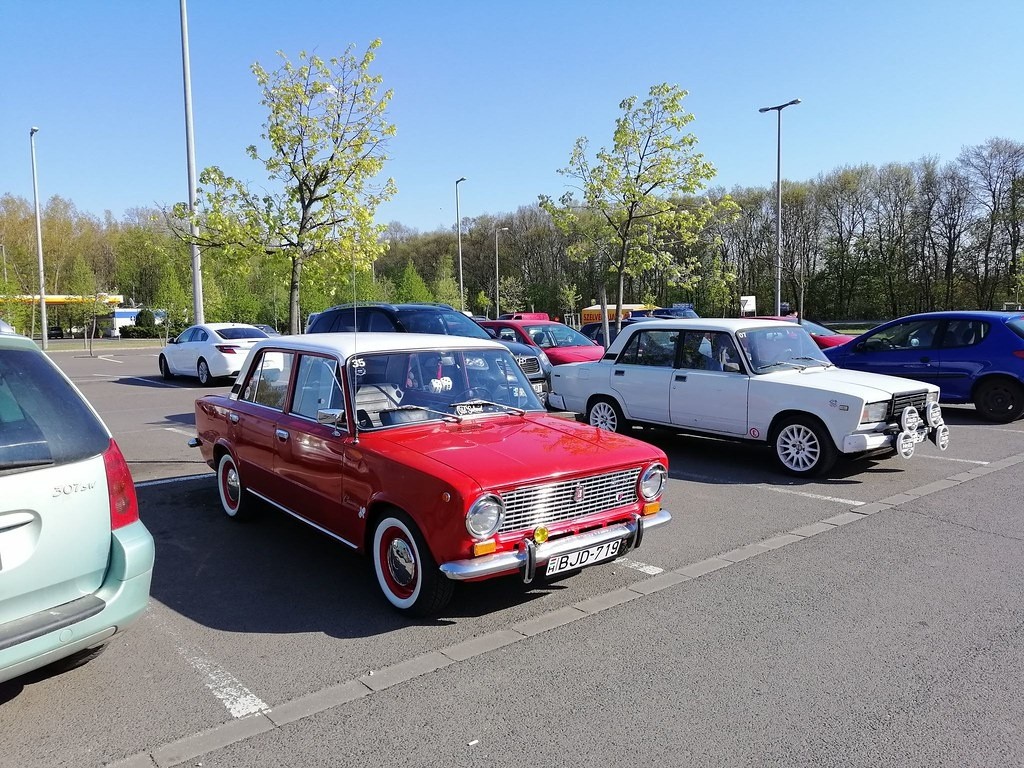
47;326;63;339
253;323;281;338
67;325;112;338
800;310;1024;426
0;319;156;685
439;302;704;367
158;322;284;388
184;331;673;620
548;316;950;482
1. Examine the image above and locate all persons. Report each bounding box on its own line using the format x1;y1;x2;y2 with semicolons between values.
642;310;653;317
626;311;632;318
786;310;798;318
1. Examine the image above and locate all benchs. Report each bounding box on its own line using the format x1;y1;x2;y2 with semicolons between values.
318;382;405;418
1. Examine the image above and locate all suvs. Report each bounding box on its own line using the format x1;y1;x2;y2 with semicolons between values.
297;303;553;411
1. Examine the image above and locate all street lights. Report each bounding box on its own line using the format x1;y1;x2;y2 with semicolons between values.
455;176;467;311
758;98;803;317
29;126;49;352
496;228;509;323
